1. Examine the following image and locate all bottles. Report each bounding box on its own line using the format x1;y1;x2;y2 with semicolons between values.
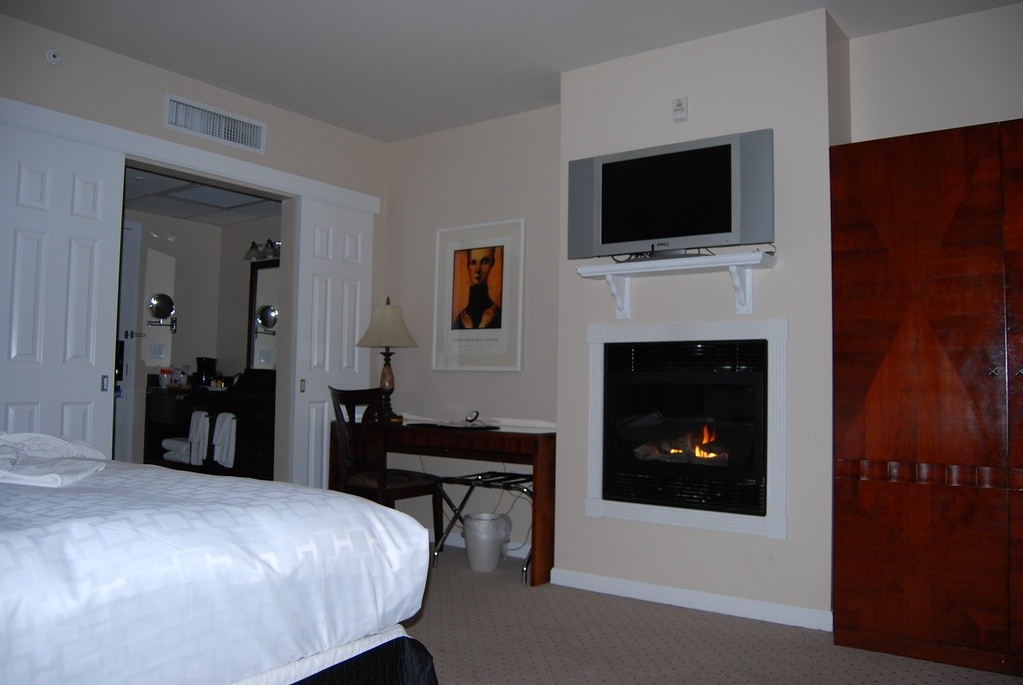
158;367;174;389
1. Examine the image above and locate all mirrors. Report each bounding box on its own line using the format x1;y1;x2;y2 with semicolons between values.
245;259;281;369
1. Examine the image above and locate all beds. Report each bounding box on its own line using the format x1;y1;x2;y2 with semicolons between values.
1;429;439;684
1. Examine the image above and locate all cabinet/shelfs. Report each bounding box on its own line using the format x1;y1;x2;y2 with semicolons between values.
143;368;276;481
829;117;1023;681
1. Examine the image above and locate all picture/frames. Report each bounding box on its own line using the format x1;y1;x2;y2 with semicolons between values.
431;217;526;373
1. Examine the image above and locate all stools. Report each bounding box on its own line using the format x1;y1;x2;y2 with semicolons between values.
434;472;536;585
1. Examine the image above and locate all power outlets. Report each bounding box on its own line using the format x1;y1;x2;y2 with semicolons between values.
260;348;272;364
150;344;165;360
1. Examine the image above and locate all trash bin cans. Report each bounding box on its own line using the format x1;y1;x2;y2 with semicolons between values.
464;514;506;572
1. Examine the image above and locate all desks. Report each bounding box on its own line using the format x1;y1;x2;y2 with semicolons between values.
327;420;556;586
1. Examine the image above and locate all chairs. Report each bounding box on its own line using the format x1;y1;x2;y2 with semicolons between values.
328;384;445;552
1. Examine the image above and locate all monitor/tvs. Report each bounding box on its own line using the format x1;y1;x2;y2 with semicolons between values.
567;128;775;262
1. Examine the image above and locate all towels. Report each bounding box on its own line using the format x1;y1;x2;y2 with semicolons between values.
188;410;210;465
162;437;191;464
212;413;237;469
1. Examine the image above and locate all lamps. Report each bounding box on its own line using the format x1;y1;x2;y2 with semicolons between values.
242;237;281;261
354;295;419;421
